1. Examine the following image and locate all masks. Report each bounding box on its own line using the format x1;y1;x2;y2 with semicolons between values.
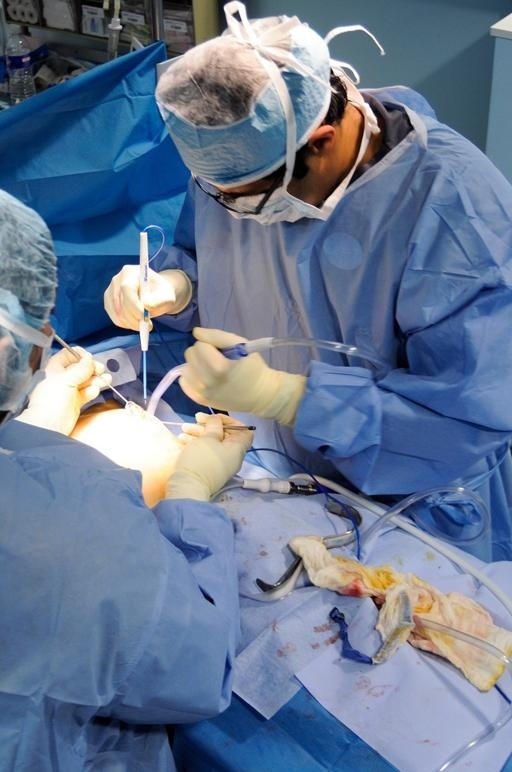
12;327;55;417
222;186;327;226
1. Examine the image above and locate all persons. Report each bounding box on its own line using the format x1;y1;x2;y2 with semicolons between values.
0;187;255;771
103;12;512;566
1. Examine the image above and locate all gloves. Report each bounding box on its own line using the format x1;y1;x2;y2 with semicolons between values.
14;345;112;436
178;327;307;428
104;264;192;333
163;411;255;501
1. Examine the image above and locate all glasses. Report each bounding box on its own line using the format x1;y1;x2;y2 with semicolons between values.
189;164;286;214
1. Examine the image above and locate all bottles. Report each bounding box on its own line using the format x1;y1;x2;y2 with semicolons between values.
5;22;38;104
